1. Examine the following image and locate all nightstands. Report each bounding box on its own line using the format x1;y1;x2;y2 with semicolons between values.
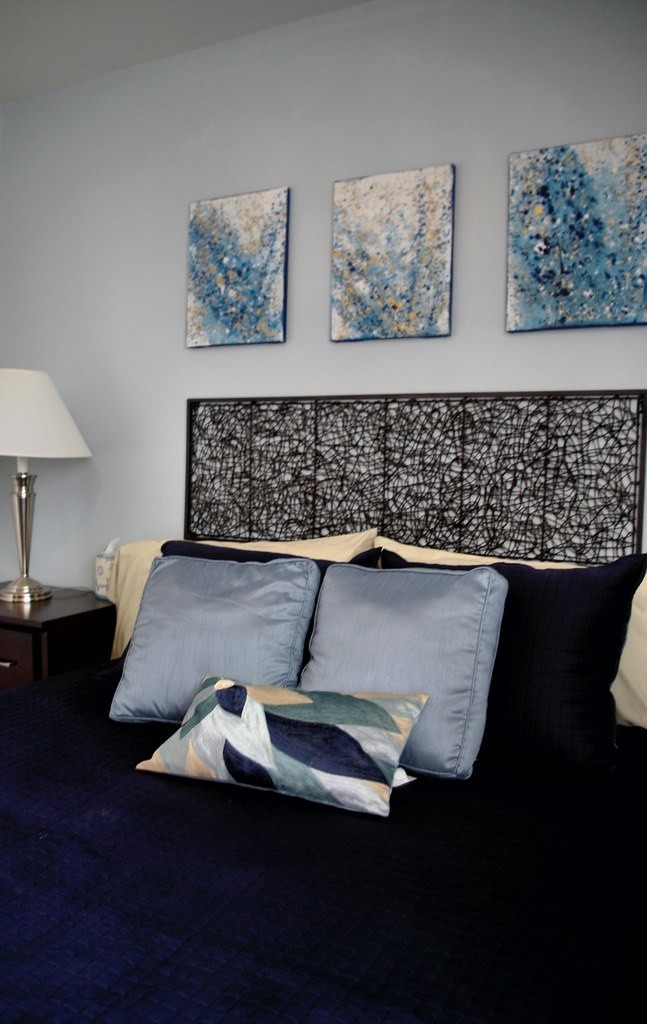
0;581;118;691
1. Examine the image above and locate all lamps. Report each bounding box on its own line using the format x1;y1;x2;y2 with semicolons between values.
0;368;95;604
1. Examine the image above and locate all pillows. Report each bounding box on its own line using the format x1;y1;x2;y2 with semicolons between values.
113;540;647;817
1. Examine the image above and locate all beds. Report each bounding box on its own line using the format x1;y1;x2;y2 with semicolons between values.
0;391;647;1024
